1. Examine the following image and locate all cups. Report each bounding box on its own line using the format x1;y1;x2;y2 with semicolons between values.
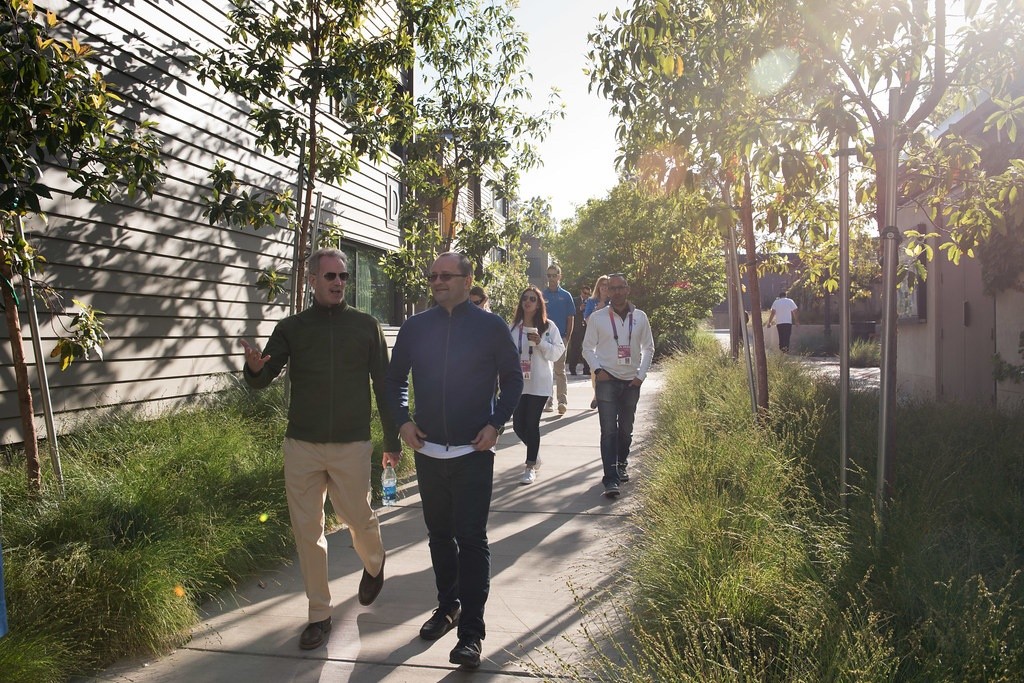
527;328;538;346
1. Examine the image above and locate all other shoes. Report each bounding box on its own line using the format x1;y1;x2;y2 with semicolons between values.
545;405;552;411
583;369;591;375
558;404;566;414
591;397;597;409
570;370;577;375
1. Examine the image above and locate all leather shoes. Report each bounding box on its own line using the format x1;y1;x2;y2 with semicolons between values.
299;618;332;648
358;551;385;607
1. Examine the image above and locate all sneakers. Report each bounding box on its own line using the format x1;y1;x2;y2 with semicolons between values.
535;455;540;470
618;462;630;482
449;634;481;667
605;482;620;496
520;467;535;484
420;606;461;640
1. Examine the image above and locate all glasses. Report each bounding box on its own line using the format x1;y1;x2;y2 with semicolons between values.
521;295;538;302
311;271;350;281
475;297;486;305
607;273;628;285
428;273;468;282
582;291;590;295
547;274;561;278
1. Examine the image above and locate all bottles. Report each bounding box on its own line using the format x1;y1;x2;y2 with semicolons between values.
380;462;396;506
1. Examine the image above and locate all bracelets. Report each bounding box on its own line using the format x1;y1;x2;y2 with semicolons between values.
488;420;505;436
594;368;602;375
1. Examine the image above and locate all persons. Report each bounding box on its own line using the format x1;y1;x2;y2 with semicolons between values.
569;286;591;377
739;308;749;340
542;265;576;414
767;292;799;353
581;275;655;497
239;247;403;651
468;287;493;314
382;252;524;667
509;287;565;484
